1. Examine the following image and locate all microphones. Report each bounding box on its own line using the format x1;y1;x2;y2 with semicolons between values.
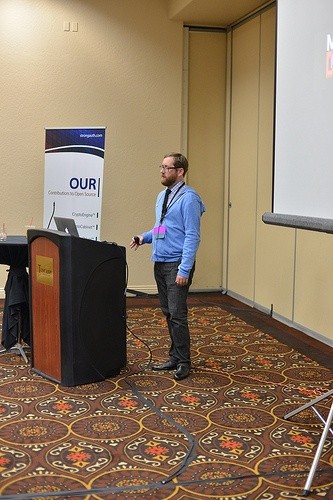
47;201;55;230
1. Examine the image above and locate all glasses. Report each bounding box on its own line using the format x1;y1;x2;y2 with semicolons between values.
159;165;183;172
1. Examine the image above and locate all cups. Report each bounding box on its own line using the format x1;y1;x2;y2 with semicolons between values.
24;225;35;238
0;223;7;242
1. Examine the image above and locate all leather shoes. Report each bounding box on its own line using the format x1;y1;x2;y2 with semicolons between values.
153;360;175;370
175;365;190;380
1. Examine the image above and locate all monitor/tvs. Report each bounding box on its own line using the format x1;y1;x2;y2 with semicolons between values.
53;217;79;237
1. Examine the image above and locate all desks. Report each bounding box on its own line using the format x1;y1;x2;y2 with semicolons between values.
0;235;32;365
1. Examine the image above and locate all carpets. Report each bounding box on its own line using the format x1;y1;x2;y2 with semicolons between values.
0;304;332;500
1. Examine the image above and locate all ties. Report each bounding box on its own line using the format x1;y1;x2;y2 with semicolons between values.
161;188;171;222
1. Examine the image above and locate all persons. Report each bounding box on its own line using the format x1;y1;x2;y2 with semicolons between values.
130;153;206;380
